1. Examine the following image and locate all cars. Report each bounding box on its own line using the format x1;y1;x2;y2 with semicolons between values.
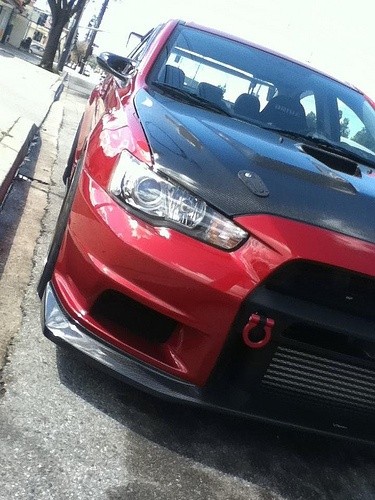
37;19;375;445
29;43;44;58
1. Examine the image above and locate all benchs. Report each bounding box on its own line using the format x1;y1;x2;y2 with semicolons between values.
179;83;263;121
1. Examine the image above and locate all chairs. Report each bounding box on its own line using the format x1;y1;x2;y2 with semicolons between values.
259;96;307;133
157;65;187;89
